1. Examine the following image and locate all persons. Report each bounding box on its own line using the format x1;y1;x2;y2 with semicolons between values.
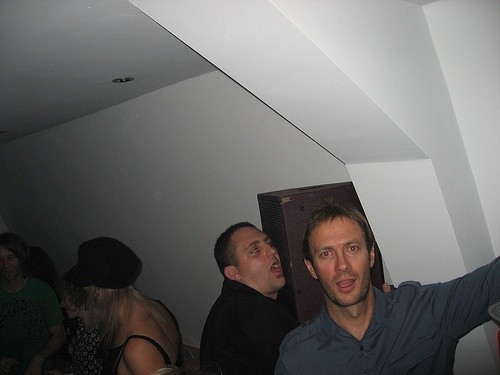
62;237;181;375
0;232;66;375
274;204;500;375
199;222;392;375
44;265;107;375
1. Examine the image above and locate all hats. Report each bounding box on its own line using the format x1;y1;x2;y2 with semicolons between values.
66;237;142;289
